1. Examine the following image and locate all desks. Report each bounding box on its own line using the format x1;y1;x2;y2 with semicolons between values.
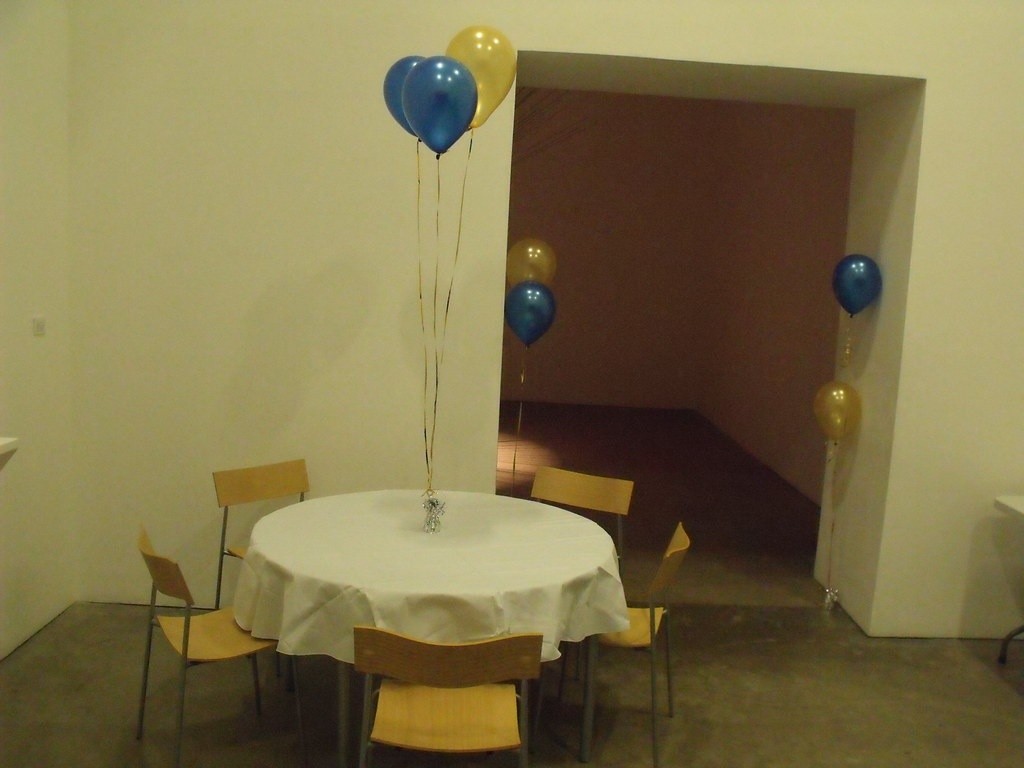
994;495;1024;665
231;490;631;768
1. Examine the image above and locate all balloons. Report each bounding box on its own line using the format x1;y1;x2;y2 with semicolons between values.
505;239;557;349
831;253;881;318
384;26;517;160
813;380;862;445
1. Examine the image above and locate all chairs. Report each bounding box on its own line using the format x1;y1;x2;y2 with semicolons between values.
529;522;690;768
353;625;544;768
531;465;634;699
212;457;311;679
134;524;308;768
